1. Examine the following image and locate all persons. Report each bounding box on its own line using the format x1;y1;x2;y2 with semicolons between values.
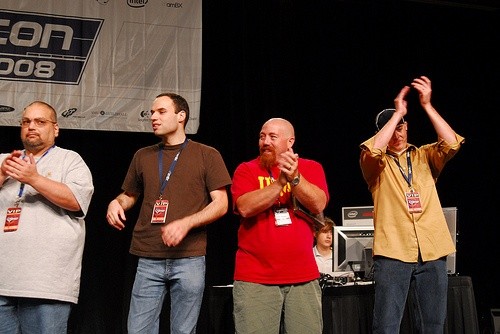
230;118;330;334
106;93;233;334
0;101;95;334
359;76;466;333
313;217;336;273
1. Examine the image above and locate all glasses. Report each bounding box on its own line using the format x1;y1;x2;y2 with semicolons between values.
19;118;57;127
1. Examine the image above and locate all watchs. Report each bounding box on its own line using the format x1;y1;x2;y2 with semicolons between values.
292;173;301;186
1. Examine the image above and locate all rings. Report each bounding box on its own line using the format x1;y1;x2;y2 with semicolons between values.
288;166;292;170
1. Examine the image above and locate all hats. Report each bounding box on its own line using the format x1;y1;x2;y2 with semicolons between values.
375;108;404;130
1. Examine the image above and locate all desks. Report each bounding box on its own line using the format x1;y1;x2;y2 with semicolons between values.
194;276;481;334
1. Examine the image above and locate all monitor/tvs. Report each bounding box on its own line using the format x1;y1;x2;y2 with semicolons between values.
332;226;376;285
